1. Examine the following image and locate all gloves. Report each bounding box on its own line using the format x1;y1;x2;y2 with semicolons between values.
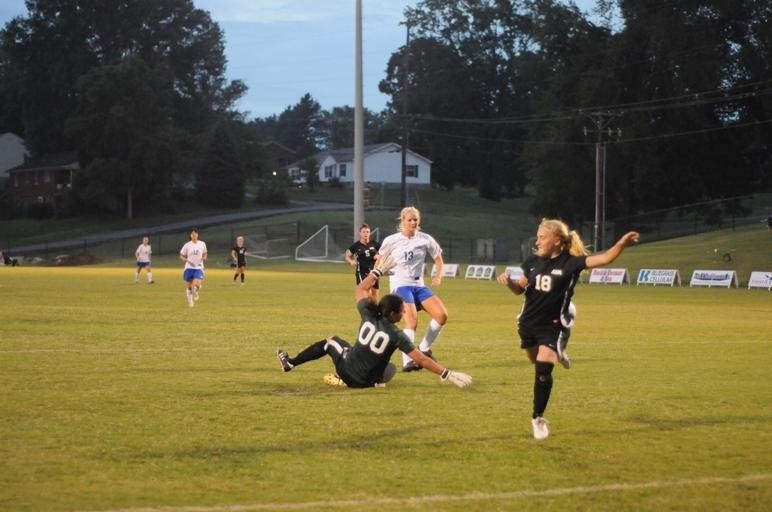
442;368;473;388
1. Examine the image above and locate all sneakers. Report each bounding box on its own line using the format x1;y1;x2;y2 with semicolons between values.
193;292;199;301
148;280;153;284
531;417;548;440
418;346;432;357
188;302;193;307
276;347;294;372
405;361;420;371
324;373;347;387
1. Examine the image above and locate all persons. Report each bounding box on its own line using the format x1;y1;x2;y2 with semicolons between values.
231;235;248;285
345;224;382;303
376;206;448;372
135;236;154;285
179;229;209;307
496;217;641;438
278;255;474;389
